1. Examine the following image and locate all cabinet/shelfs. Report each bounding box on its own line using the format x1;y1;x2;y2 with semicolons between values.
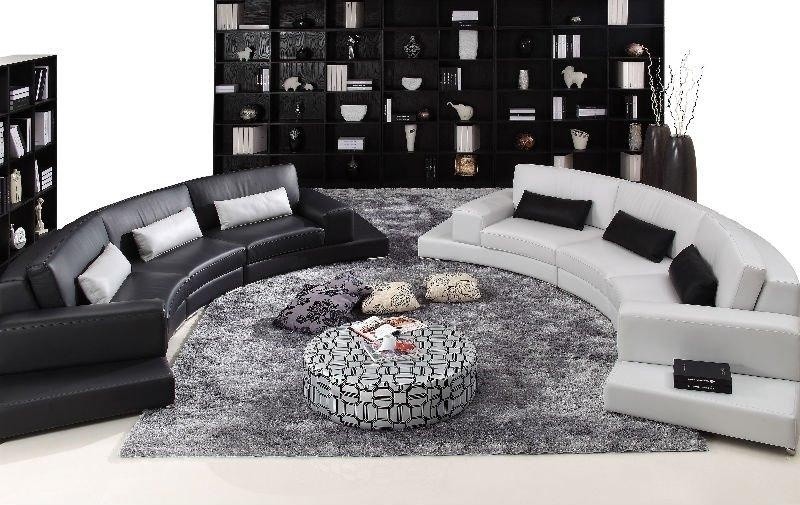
213;0;665;185
0;54;58;276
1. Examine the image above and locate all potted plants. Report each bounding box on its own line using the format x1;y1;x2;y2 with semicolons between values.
664;48;705;201
642;45;670;188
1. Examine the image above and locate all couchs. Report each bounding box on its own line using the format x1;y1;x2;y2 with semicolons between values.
417;162;798;456
0;165;388;439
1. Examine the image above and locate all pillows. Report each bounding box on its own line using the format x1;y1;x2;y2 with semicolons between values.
214;186;292;231
603;210;675;264
513;164;617;230
614;180;701;259
513;190;592;231
132;206;204;262
186;164;300;231
25;215;110;308
100;185;195;259
695;213;766;310
78;242;132;305
668;243;718;304
271;271;481;334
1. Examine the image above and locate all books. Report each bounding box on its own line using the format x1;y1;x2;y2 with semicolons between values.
673;359;732;394
386;99;410;123
607;0;630;26
552;34;581;59
239;24;270;30
262;68;269;92
232;127;267;156
553;97;562;119
442;67;462;91
633;95;638;120
216;84;238;93
345;2;361;29
616;60;645;90
620;152;642;182
454;123;480;154
347;316;428;363
577;107;605;117
553;154;573;169
216;4;237;31
327;65;372;92
0;65;52;214
508;108;535;121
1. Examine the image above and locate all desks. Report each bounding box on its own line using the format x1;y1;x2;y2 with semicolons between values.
303;318;478;430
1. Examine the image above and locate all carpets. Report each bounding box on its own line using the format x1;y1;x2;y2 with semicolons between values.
119;188;711;456
